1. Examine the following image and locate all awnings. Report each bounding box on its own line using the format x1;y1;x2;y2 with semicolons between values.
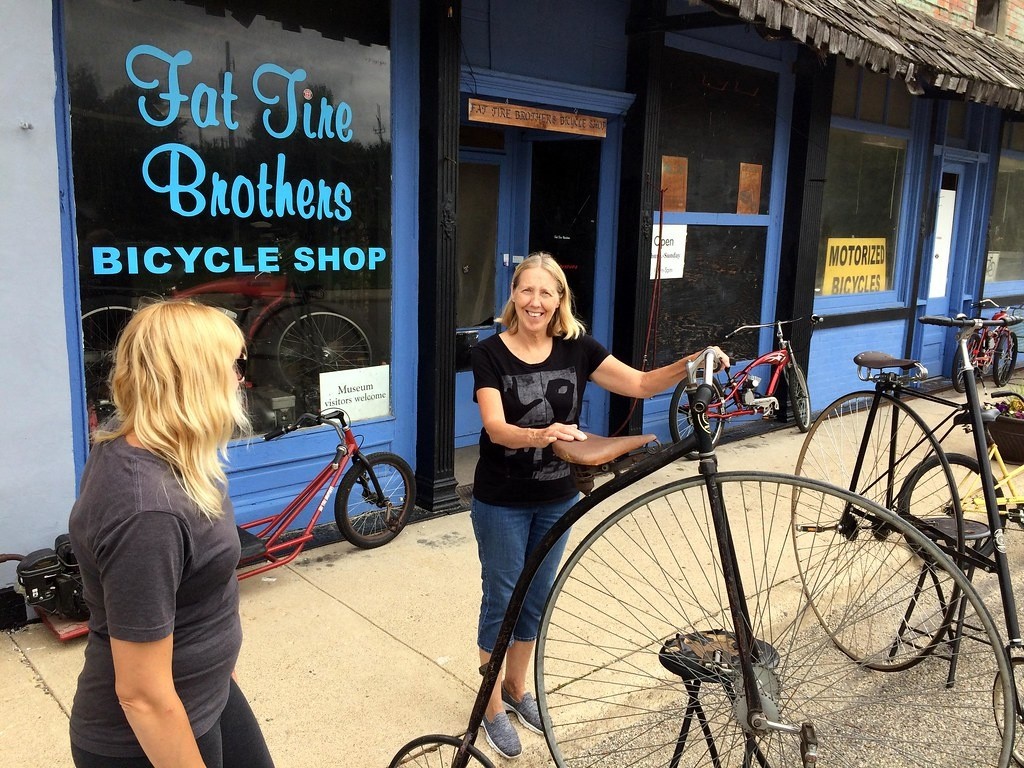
722;0;1024;103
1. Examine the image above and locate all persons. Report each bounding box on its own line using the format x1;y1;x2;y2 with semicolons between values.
69;300;276;768
469;254;731;762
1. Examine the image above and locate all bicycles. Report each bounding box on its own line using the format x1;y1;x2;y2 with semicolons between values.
17;405;417;640
387;343;1016;768
900;409;1024;569
790;315;1024;768
952;298;1024;393
670;312;823;460
82;194;373;405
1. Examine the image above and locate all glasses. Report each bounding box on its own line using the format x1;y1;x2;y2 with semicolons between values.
234;351;247;383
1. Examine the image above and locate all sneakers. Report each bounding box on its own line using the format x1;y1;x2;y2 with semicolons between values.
501;680;554;735
480;712;522;759
